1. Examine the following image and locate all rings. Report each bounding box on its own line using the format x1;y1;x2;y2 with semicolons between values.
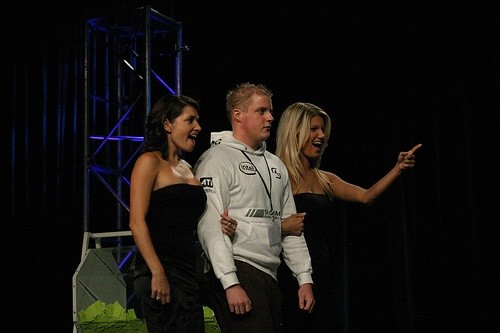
228;222;232;227
156;296;161;299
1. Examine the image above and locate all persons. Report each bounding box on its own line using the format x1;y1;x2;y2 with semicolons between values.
192;82;315;333
129;95;237;333
275;102;421;332
